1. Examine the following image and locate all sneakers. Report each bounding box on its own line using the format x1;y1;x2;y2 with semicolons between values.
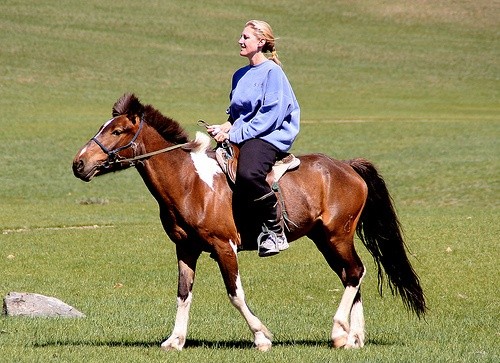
261;233;290;251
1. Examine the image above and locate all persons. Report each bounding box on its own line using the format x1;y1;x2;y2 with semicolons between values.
205;19;301;252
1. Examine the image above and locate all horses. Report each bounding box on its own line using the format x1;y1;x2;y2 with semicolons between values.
72;92;431;352
